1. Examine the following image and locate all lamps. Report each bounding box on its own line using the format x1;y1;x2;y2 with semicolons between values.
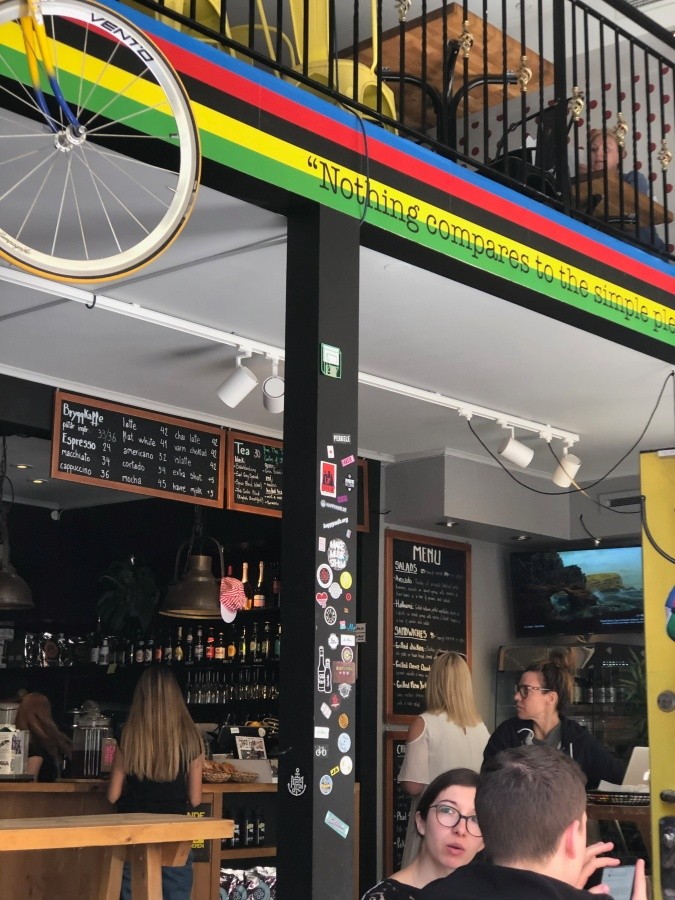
552;438;582;489
0;432;35;609
496;418;536;469
216;349;260;408
157;503;228;621
262;349;285;415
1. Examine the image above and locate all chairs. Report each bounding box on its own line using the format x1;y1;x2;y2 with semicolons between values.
476;96;580;217
258;1;400;138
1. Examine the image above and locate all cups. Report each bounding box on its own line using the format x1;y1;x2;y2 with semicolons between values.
70;717;112;779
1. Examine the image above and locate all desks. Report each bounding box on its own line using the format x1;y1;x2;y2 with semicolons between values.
583;801;656;870
569;168;675;241
0;810;237;900
335;2;555;162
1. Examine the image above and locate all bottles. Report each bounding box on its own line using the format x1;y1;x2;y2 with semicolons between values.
228;804;266;848
228;559;278;609
30;621;282;666
180;670;279;703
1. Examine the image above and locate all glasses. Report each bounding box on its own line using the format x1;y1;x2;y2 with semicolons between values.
427;805;485;838
514;684;552;698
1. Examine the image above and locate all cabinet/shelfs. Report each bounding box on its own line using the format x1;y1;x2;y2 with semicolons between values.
65;604;282;739
216;780;360;900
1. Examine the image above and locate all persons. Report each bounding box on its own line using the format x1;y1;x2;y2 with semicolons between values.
394;652;491;872
587;128;667;254
478;655;628;789
416;741;647;900
360;768;487;900
14;691;63;784
105;663;203;900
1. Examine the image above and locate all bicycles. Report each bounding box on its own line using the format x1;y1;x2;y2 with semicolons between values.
0;0;204;285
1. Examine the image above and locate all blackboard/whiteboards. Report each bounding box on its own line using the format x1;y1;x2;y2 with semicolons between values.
382;527;473;722
49;391;227;509
382;730;410;879
224;431;370;532
